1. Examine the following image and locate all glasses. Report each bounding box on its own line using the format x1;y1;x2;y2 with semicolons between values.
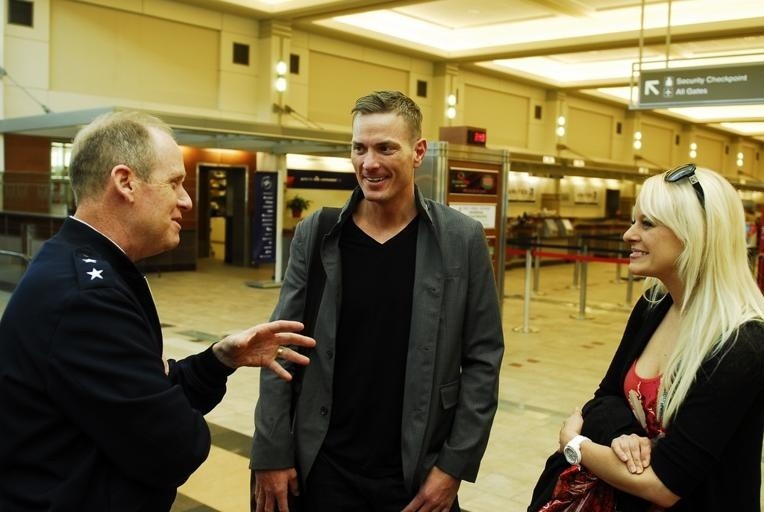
665;162;705;210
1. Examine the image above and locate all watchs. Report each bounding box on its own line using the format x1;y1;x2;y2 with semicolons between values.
563;434;592;467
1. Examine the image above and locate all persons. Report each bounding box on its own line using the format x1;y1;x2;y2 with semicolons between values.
559;167;764;512
249;91;505;512
0;113;317;512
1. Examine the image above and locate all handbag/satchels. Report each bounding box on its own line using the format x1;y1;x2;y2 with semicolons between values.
251;461;297;512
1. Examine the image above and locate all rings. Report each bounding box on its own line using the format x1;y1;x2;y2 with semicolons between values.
277;346;284;359
